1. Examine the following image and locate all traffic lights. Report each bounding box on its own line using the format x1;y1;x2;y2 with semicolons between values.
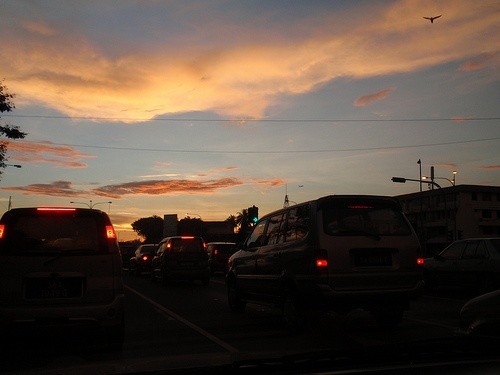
248;208;259;223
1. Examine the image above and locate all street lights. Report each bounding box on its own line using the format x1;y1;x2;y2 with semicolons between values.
421;170;457;241
70;200;112;209
186;213;202;221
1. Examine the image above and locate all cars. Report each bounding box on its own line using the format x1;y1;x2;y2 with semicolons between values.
129;243;161;277
429;237;500;320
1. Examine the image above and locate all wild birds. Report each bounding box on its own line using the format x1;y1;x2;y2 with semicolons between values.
423;15;442;24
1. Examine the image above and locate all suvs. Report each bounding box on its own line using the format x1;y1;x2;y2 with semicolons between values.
0;208;126;354
226;195;425;335
207;242;236;275
152;236;211;288
